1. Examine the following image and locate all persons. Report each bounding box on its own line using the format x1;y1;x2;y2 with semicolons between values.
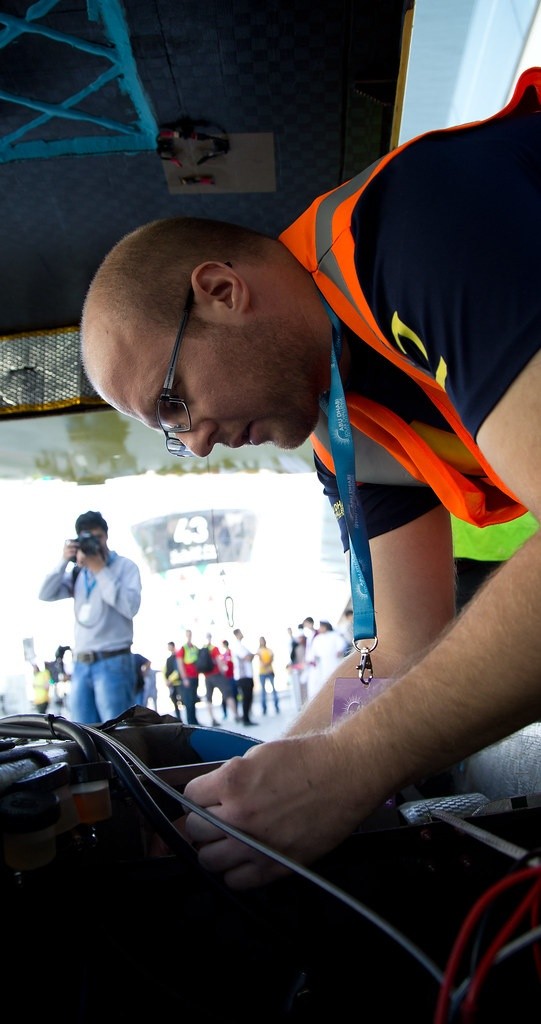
35;510;141;728
284;608;357;705
0;628;280;735
77;63;541;864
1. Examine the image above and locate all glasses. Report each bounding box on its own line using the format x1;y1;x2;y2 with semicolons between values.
156;261;234;459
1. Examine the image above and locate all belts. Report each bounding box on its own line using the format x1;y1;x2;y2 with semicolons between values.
73;646;130;662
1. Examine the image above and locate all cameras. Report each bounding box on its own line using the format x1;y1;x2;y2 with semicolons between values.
72;531;102;555
